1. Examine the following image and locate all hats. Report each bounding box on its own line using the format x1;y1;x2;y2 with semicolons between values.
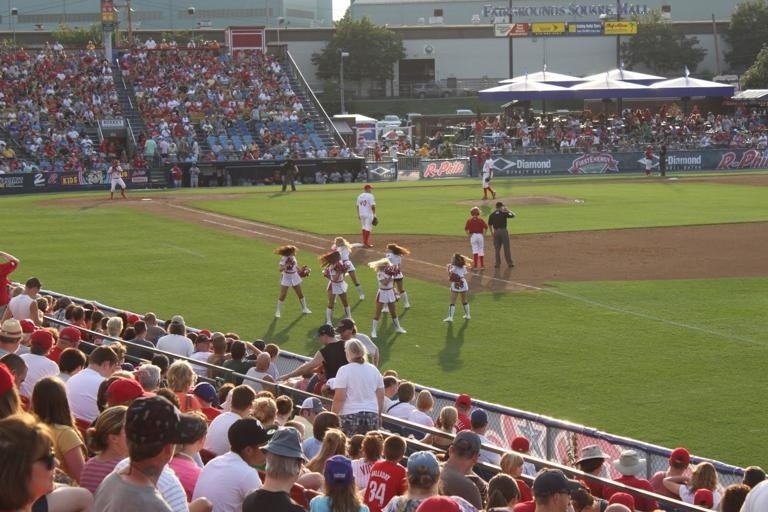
193;382;216;401
107;379;157;406
471;409;487;424
125;396;201;442
335;319;353;332
407;451;438;475
608;492;634;512
671;448;689;463
228;418;277;448
171;314;183;325
576;445;609;464
453;430;481;451
1;318;81;347
365;186;373;189
512;437;529;452
496;203;503;207
533;469;579;492
301;397;327;411
456;393;471;408
258;426;307;462
323;455;353;484
613;450;646;475
694;489;713;507
315;325;335;336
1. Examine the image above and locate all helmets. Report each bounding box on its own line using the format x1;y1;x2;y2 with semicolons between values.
470;207;479;215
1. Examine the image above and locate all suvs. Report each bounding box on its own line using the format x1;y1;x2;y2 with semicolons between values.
413;82;452;98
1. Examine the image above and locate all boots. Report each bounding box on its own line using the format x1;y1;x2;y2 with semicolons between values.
345;307;354;323
356;285;364;300
299;297;311;314
381;304;389;312
371;320;378;337
326;308;333;327
392;318;406;333
444;304;455;321
275;300;284;317
399;290;410;308
462;303;471;319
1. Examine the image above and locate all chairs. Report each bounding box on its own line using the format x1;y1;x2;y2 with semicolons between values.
464;113;768;156
4;44;337;169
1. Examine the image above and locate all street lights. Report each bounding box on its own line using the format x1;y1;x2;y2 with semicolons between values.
10;8;18;54
339;51;349;112
188;6;196;40
275;15;283;47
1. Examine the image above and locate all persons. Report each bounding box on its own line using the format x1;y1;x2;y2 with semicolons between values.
487;201;517;269
330;237;366;301
643;145;653;176
271;244;312;318
352;102;768;165
2;250;767;510
480;152;496;200
384;243;412;310
355;184;379;248
657;141;666;176
463;206;489;270
2;37;352;191
106;159;128;200
